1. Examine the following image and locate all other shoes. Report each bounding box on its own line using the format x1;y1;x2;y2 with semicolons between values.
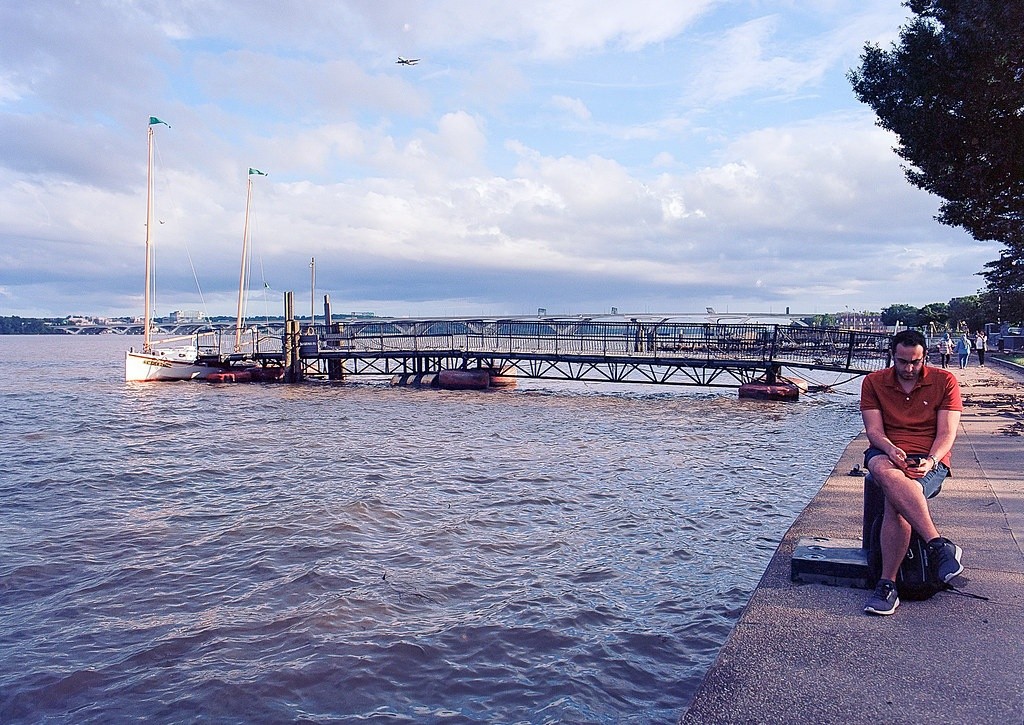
981;365;984;368
959;367;962;369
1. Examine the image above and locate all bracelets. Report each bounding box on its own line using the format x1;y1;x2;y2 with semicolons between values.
926;454;938;470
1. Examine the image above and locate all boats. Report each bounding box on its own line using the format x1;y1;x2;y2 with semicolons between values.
124;114;263;387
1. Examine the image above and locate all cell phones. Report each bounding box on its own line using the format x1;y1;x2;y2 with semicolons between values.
905;457;921;468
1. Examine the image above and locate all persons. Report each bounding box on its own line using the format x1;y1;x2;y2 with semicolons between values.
859;329;965;616
936;328;988;370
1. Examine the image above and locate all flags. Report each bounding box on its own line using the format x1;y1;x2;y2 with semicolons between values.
150;116;172;129
249;168;269;178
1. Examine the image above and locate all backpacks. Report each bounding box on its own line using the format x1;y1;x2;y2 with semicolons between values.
870;513;946;599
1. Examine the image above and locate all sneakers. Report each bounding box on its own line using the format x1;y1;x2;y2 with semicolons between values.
864;578;900;615
927;536;964;583
